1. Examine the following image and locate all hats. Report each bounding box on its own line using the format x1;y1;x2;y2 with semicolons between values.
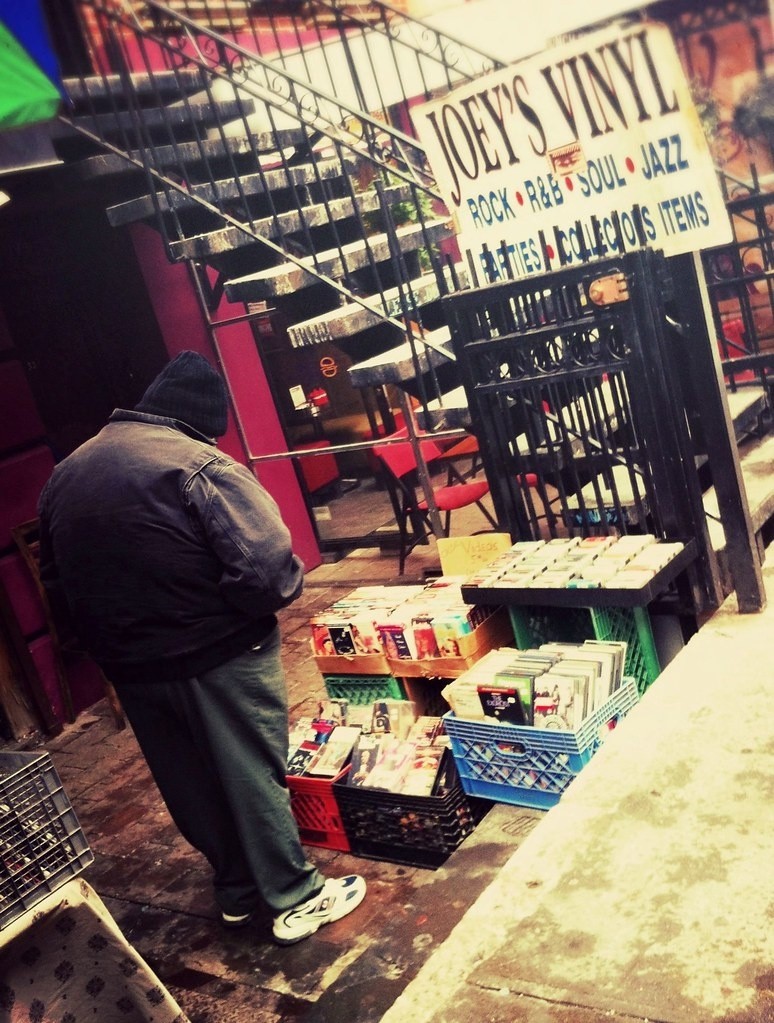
134;351;228;437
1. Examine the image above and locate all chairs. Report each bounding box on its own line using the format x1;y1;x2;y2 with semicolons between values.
371;420;498;575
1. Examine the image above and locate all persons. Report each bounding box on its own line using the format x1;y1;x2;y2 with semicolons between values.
383;633;400;659
287;753;311;774
39;353;368;942
441;639;461;657
534;684;574;719
372;703;391;733
351;751;372;787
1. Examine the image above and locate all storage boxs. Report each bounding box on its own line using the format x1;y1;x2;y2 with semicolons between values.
286;762;353;851
309;637;390;675
388;616;492;678
330;772;483;871
510;605;661;698
442;677;640;811
0;751;95;931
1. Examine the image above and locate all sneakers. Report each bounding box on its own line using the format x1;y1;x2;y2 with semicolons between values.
222;911;252;927
272;874;367;940
